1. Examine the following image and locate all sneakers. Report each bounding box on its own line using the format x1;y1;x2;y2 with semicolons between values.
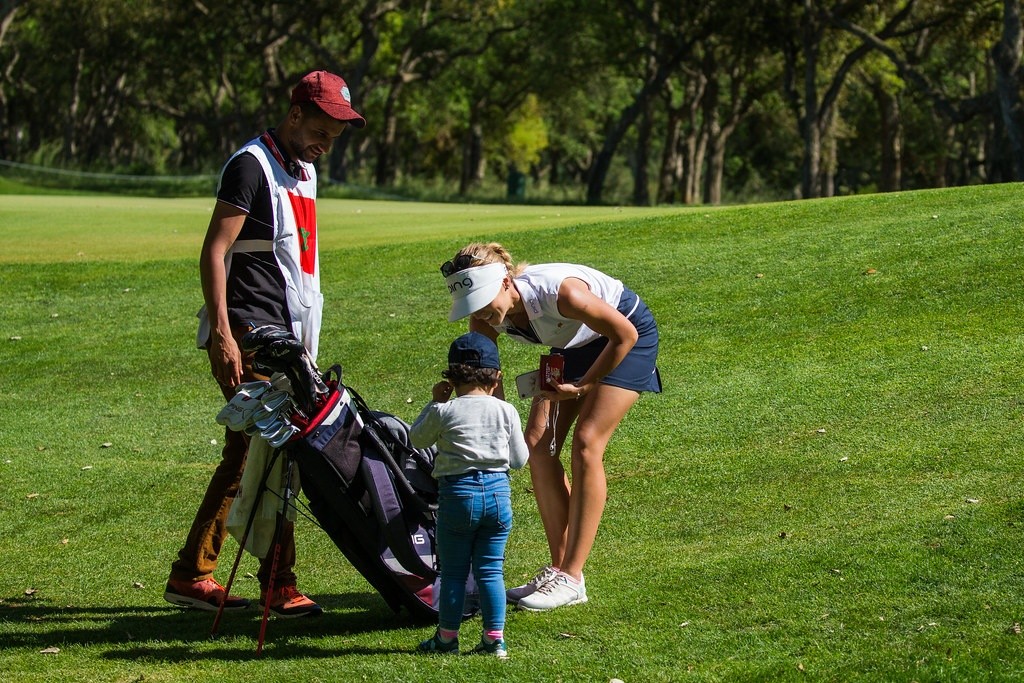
517;571;588;612
479;630;507;658
505;567;558;602
163;578;248;611
416;626;458;653
259;584;322;620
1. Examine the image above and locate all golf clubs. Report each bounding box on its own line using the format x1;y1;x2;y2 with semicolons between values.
252;408;295;429
243;419;260;436
260;390;311;423
266;426;300;448
260;422;288;438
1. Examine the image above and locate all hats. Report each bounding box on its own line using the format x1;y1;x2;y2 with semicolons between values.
291;71;366;129
446;263;508;321
448;331;501;371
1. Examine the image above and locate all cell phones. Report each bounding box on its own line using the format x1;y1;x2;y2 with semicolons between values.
516;370;539;400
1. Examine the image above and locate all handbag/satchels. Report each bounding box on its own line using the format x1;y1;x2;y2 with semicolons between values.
295;363;482;625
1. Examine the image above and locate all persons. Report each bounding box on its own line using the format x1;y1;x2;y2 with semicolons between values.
409;331;530;657
163;70;365;619
441;241;659;613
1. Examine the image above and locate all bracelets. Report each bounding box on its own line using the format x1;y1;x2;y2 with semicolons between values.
574;381;582;401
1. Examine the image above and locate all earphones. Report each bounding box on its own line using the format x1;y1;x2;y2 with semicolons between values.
550;441;553;450
551;447;556;457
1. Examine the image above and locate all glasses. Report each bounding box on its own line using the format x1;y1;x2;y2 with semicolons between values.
440;255;494;278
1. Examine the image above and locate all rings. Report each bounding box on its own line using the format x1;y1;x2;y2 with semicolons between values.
216;375;223;381
